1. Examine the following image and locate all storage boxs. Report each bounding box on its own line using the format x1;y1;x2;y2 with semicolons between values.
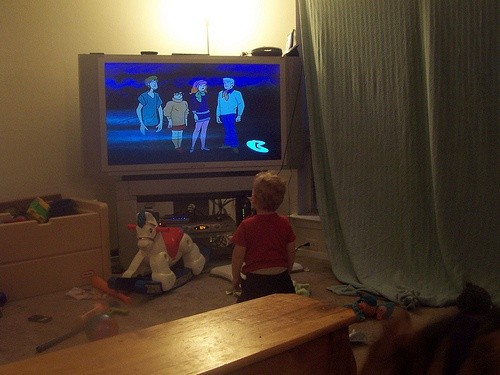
0;192;111;303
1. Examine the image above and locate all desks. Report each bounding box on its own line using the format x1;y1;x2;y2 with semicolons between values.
1;293;360;375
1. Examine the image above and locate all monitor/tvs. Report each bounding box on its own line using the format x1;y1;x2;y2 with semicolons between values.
78;54;305;181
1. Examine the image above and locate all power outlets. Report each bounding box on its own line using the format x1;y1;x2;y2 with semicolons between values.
304;239;316;249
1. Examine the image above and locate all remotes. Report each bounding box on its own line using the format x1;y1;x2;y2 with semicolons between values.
141;51;158;55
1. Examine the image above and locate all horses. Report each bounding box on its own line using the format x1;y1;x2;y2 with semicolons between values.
107;210;210;293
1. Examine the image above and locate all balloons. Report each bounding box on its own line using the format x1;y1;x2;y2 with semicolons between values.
85;314;121;341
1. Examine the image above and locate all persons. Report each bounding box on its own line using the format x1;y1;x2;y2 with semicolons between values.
228;172;296;303
